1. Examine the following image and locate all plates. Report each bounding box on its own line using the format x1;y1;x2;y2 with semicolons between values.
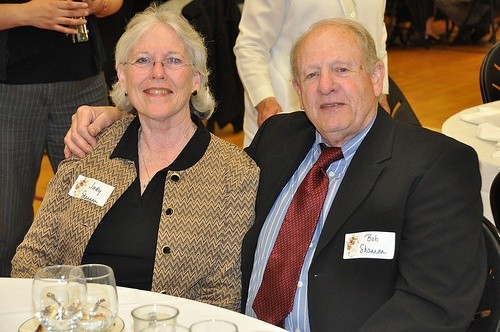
18;303;125;332
475;124;500;142
459;106;496;124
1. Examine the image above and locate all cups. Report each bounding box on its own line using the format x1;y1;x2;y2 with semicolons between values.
70;264;118;332
132;303;189;332
31;264;87;332
189;319;238;332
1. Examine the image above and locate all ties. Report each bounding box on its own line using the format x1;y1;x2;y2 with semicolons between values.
252;143;344;324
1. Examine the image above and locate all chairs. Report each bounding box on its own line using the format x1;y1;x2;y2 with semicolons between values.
464;40;500;332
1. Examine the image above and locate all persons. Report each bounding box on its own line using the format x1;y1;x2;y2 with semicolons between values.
0;0;125;279
234;0;391;149
64;18;487;332
11;9;260;313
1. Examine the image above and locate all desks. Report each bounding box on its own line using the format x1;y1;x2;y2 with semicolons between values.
0;278;291;332
441;101;500;226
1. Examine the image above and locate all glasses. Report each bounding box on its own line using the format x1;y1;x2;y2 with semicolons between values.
120;51;195;71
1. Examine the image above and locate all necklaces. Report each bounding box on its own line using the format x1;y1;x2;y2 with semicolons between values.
138;123;197;180
